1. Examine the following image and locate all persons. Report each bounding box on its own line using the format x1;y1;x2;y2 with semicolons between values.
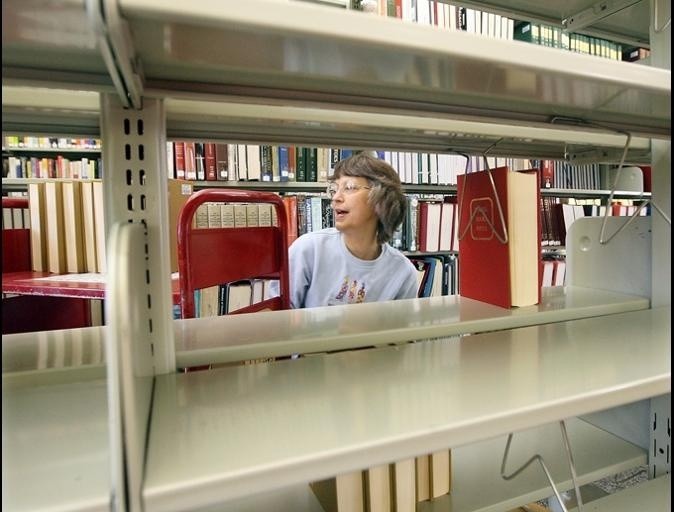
268;152;421;359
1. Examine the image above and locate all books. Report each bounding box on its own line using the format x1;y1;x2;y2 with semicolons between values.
308;445;452;511
166;140;653;319
2;136;107;327
311;1;514;40
514;22;651;63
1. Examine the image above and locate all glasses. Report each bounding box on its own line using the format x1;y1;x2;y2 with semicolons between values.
325;181;373;197
